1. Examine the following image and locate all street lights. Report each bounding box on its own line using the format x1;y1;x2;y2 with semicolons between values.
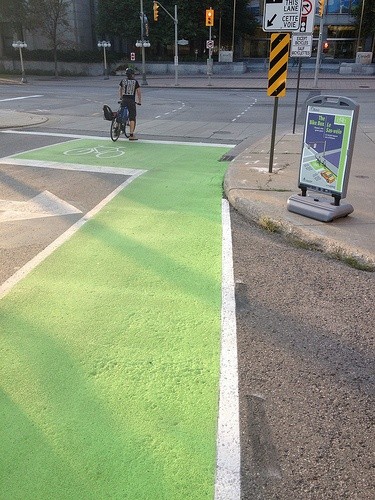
136;40;151;85
98;41;111;80
12;40;28;84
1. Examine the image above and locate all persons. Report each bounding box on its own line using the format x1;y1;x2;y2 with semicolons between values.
114;68;142;140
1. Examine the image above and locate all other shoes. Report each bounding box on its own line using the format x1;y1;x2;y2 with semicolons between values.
129;136;138;140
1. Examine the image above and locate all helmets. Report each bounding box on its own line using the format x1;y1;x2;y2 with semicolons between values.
125;68;134;76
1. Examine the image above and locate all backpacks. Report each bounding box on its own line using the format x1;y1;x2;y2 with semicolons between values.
103;105;113;120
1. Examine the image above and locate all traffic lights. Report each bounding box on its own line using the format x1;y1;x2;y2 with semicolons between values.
154;3;159;22
318;0;325;16
205;9;214;27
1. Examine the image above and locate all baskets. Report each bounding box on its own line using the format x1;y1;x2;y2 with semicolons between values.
103;111;117;121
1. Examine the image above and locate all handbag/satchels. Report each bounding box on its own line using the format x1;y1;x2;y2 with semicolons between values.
116;107;129;124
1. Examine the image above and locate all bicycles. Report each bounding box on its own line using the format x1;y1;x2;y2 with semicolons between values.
110;100;141;142
